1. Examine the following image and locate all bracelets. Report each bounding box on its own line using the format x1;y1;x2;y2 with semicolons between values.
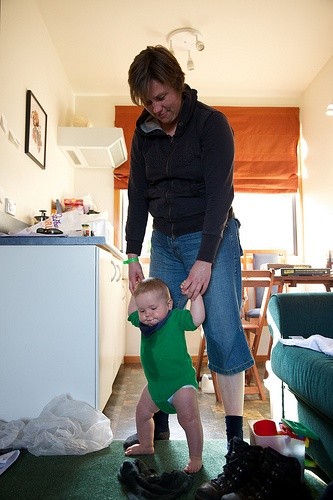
123;258;139;265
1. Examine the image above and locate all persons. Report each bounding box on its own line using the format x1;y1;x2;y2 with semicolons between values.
125;278;205;473
123;45;255;463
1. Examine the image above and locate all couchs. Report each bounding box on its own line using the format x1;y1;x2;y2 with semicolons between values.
267;293;333;459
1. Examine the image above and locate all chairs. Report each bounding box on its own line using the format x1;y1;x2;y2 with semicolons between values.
197;268;274;403
243;250;287;378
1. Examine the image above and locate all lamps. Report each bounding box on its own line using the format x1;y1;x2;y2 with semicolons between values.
166;29;207;71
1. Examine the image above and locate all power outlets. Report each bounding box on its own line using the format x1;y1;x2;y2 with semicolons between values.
5;197;15;216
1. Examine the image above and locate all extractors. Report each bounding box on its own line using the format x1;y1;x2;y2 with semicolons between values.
57;127;129;169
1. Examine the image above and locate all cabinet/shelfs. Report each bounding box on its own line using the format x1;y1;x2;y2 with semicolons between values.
0;245;129;421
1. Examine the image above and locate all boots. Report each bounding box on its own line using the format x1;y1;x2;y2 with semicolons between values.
195;436;311;500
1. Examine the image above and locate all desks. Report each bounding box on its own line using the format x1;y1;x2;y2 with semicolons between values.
267;275;333;293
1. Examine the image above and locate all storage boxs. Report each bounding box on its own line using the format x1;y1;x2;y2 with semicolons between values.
92;219;114;245
247;420;305;470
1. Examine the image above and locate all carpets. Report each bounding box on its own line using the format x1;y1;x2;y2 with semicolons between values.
0;440;327;500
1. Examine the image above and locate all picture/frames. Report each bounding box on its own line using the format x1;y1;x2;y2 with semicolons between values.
25;90;47;170
